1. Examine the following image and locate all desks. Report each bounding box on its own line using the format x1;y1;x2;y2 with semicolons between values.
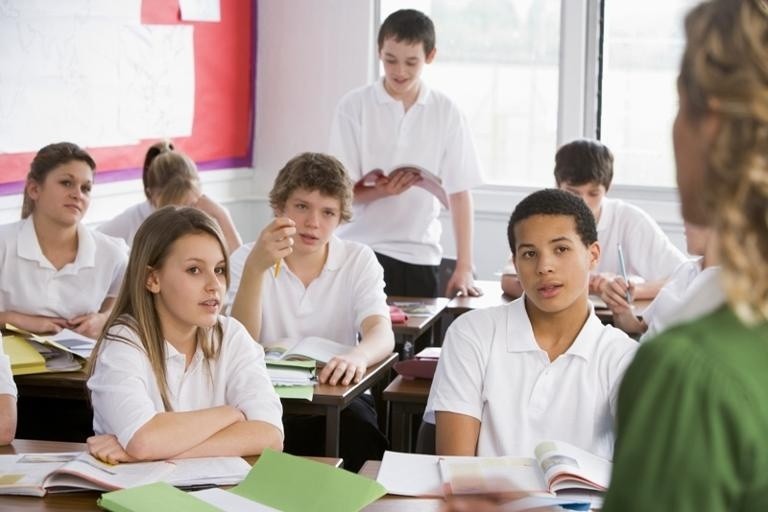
283;352;402;470
351;456;450;511
2;433;344;510
382;345;441;455
447;281;652;322
14;357;93;406
386;295;449;362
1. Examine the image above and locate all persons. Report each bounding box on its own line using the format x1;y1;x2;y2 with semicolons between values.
329;8;484;299
421;187;643;464
221;153;396;386
500;137;689;300
437;0;767;512
94;139;243;255
0;330;21;446
602;210;729;343
0;142;129;340
87;204;285;465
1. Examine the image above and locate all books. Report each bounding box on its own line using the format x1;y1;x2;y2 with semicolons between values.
0;449;172;498
434;438;612;501
262;333;350;368
355;162;450;209
163;455;251;487
56;337;95;351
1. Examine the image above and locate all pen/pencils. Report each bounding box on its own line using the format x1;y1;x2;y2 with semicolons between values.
617;243;630;303
274;258;281;279
